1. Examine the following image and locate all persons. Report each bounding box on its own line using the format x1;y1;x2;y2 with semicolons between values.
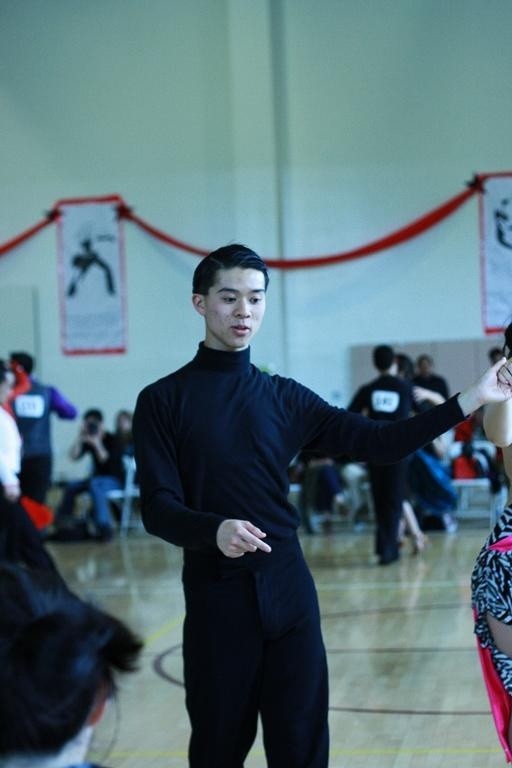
0;494;144;768
0;352;76;541
287;346;507;565
468;321;512;768
129;241;512;766
43;408;140;541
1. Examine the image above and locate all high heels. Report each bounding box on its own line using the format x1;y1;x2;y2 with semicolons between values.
411;532;430;556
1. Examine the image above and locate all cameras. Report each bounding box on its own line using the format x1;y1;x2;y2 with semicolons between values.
86;422;99;437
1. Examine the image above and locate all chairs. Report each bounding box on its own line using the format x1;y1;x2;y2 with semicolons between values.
445;439;506;535
